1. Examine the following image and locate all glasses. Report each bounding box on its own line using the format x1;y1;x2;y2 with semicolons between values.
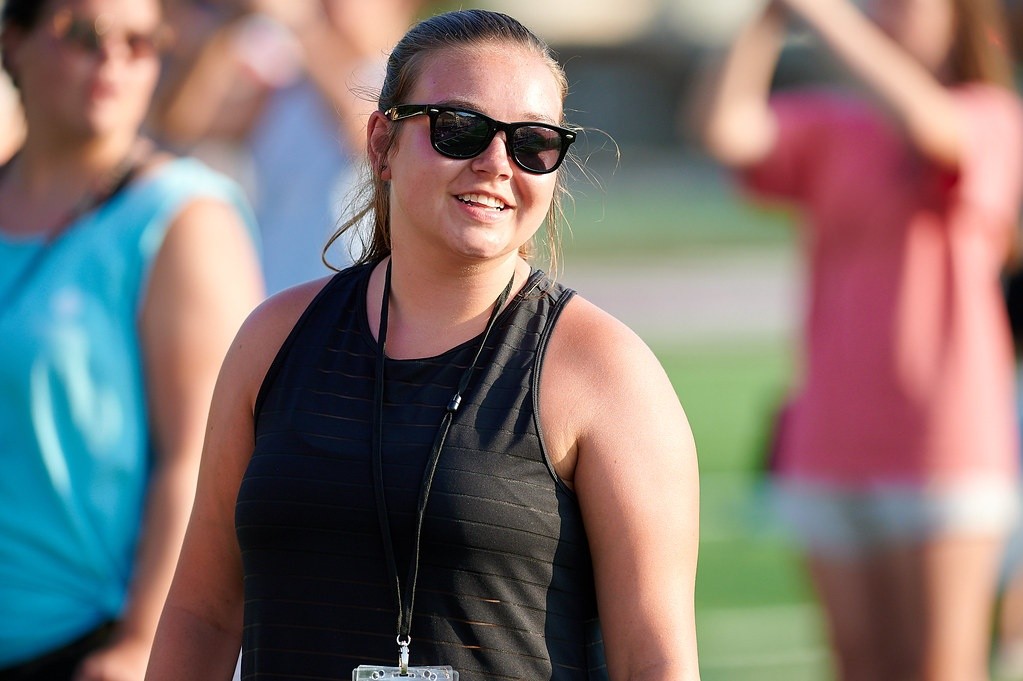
24;15;169;61
385;105;580;175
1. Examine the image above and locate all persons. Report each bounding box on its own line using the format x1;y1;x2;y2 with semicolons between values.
0;0;413;680
142;10;701;680
702;0;1022;680
